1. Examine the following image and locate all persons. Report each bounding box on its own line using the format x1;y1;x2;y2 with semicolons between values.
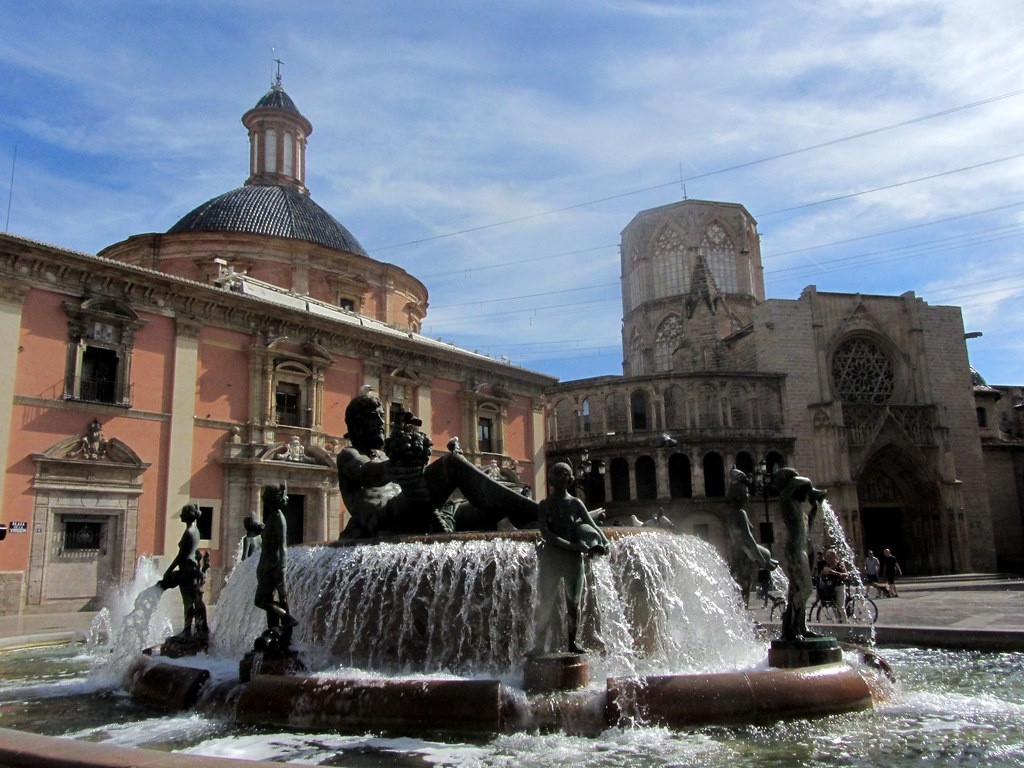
726;481;768;612
445;437;463;455
334;391;538;534
253;483;299;648
754;543;903;616
160;502;210;641
242;515;262;561
779;476;823;641
523;461;610;657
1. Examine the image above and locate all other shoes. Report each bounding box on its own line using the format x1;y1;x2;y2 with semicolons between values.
890;593;894;597
894;593;899;597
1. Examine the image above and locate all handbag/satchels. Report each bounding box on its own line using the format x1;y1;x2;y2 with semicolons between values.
816;575;836;602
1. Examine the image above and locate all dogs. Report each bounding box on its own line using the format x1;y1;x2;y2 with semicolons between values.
872;583;894;599
